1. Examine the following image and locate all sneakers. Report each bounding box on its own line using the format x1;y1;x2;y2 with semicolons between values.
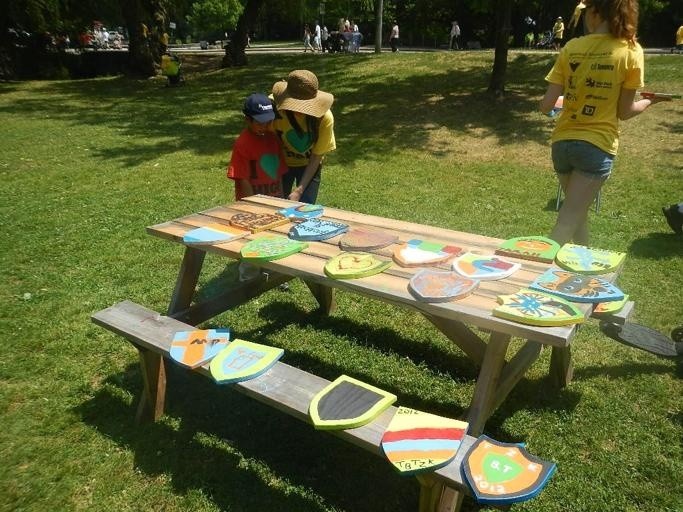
239;262;259;282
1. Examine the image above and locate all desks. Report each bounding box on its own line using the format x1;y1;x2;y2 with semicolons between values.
147;192;625;511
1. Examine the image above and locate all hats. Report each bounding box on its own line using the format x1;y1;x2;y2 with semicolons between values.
242;94;276;123
273;70;335;119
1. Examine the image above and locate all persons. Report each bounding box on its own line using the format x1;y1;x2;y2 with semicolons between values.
302;17;358;52
73;27;124;50
269;70;334;204
226;91;287;281
389;19;400;52
448;21;461;50
525;16;566;50
676;26;683;49
538;0;671;246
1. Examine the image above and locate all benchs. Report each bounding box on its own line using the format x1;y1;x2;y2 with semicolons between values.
90;297;512;511
547;299;637;387
